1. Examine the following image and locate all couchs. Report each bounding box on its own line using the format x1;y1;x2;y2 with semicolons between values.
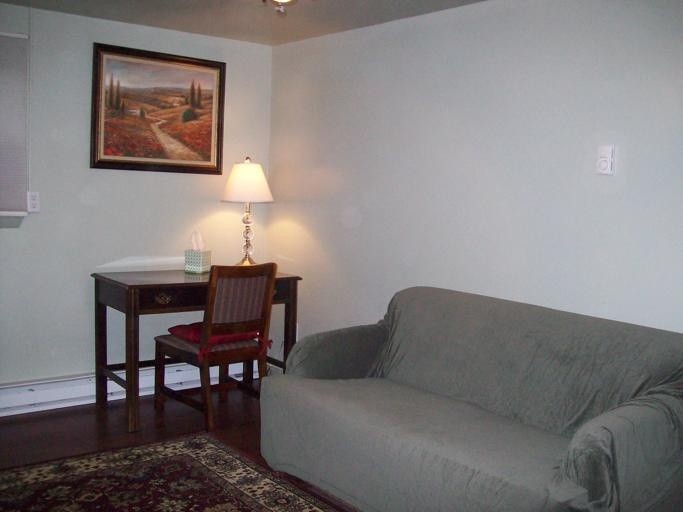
257;287;683;512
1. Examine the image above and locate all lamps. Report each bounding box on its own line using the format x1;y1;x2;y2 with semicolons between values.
218;154;276;275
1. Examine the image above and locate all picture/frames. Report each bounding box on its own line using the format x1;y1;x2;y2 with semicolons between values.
87;38;228;176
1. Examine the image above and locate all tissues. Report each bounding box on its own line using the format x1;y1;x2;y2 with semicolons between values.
184;217;212;274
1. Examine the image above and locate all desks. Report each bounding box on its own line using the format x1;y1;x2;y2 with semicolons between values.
89;267;302;432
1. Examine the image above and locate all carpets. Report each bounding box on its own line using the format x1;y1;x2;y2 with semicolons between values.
0;425;355;511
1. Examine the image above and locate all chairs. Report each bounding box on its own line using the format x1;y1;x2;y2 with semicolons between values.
147;260;281;435
